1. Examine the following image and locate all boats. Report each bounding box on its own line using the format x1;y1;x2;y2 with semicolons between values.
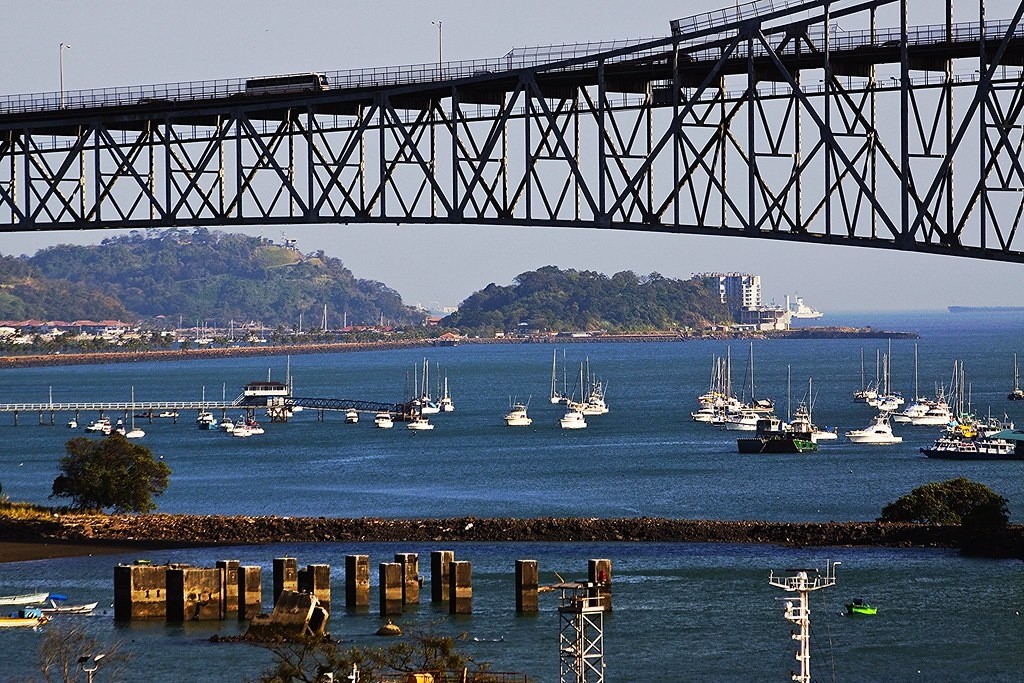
132;560;151;565
374;410;392;426
843;407;904;444
849;338;1024;459
196;382;265;438
502;394;532;426
0;606;53;628
378;419;394;429
377;623;403;634
159;411;179;418
844;598;878;615
406;416;435;430
736;418;818;453
345;408;359;423
86;415;126;436
38;595;99;615
0;588;50;605
67;418;77;429
560;408;588;429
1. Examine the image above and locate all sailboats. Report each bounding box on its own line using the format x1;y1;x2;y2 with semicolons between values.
409;355;455;414
1007;351;1024;402
126;385;146;438
548;347;610;416
689;341;838;439
